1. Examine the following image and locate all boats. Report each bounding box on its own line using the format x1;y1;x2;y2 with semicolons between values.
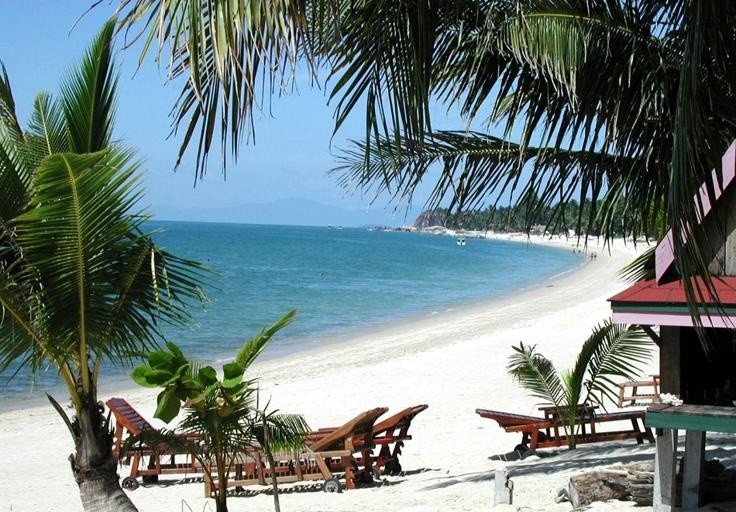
455;238;468;247
